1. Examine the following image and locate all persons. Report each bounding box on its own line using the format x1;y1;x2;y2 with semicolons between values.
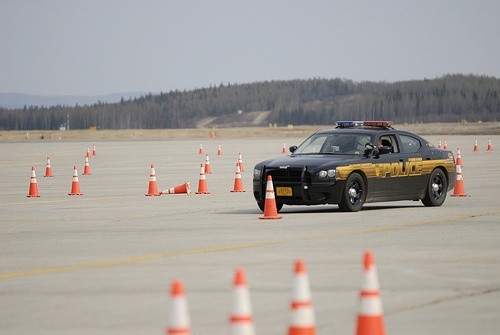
367;135;391;155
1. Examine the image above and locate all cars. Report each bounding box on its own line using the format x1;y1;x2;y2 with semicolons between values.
253;120;457;213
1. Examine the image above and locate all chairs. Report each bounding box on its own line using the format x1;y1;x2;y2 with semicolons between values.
382;139;394;154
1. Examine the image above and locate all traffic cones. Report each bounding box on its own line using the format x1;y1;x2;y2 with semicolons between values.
161;181;189;195
436;139;448;149
68;165;83;195
473;140;479;152
145;164;161;196
195;129;247;194
26;166;40;197
224;269;256;335
449;147;469;197
355;251;385;335
164;278;192;335
42;144;96;177
259;176;284;219
486;138;494;151
280;143;286;154
284;259;318;335
